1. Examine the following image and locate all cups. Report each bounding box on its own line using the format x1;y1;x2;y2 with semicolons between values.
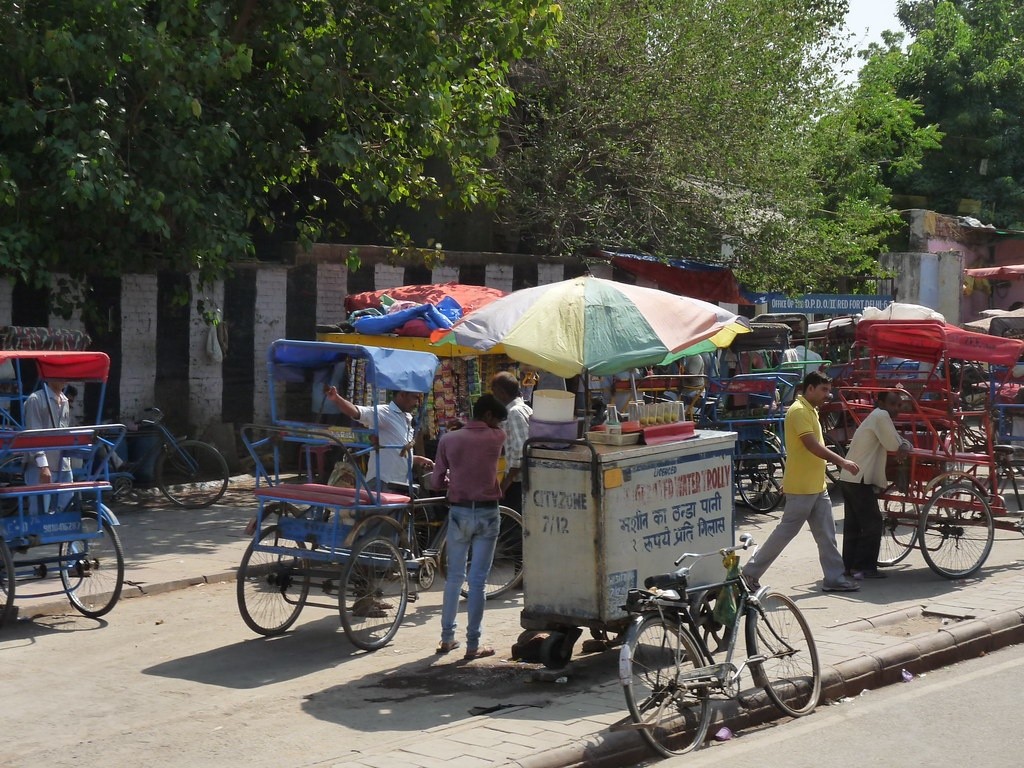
648;403;657;425
670;401;679;424
656;403;665;425
676;401;685;422
637;399;644;406
664;402;672;424
640;404;649;427
628;401;639;428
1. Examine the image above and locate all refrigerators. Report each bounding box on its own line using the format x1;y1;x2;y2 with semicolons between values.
521;429;739;622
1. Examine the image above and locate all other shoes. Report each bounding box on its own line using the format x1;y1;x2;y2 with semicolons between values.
850;569;887;578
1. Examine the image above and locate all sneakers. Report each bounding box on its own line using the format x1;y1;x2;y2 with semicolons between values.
741;571;761;592
822;581;860;592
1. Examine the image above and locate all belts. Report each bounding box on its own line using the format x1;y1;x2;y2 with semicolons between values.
450;500;499;508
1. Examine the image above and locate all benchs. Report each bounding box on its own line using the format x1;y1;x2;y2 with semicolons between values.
0;481;115;499
253;481;410;508
822;401;855;412
722;405;791;419
901;398;948;411
971;380;1023;402
888;447;993;466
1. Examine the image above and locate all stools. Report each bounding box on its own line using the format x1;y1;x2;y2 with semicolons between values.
298;444;334;481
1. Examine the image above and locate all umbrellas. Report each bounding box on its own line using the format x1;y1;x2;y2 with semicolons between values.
428;271;754;439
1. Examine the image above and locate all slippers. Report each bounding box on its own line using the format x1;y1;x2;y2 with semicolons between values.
436;639;460;653
464;644;495;659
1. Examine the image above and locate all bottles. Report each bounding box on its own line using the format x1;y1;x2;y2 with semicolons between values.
605;404;620;434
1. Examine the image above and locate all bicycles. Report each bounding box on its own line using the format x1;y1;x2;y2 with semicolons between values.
607;532;824;756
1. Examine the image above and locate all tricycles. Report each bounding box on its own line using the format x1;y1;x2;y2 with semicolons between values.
628;286;1024;512
0;347;126;627
831;314;1024;581
233;337;534;651
0;382;231;509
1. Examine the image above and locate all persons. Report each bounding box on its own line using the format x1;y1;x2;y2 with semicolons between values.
739;370;861;592
491;371;535;591
428;394;510;660
25;378;93;579
61;385;79;427
839;386;915;580
321;382;436;618
775;333;825;407
593;345;741;415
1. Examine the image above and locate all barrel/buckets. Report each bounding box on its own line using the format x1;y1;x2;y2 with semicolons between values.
528;413;578;448
532;389;574;422
